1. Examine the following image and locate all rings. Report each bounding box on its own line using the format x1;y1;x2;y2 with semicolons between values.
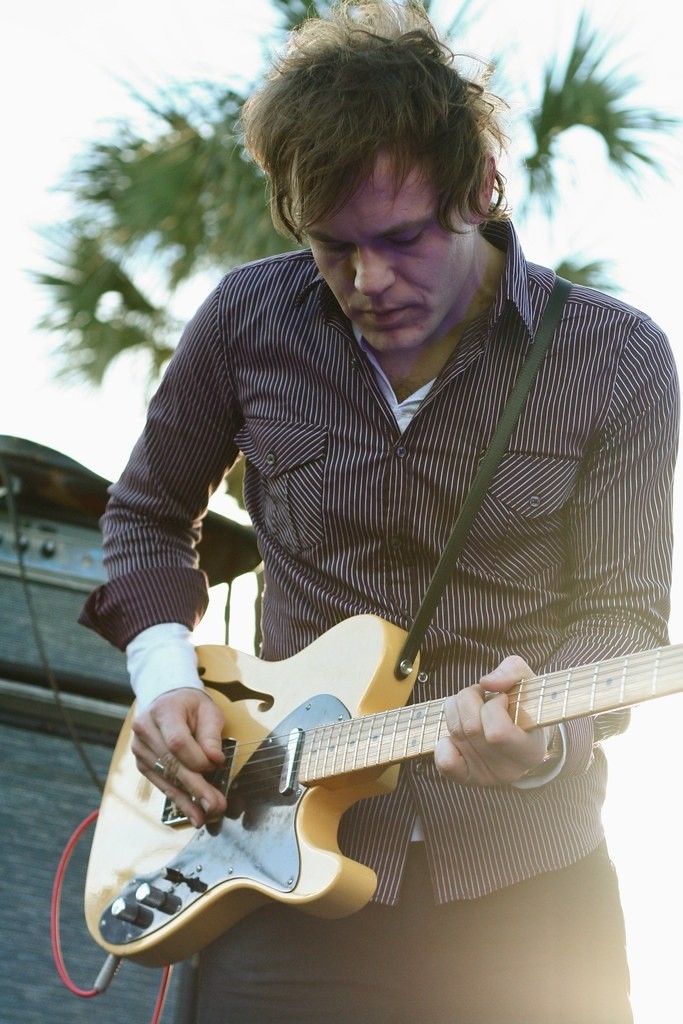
153;758;165;775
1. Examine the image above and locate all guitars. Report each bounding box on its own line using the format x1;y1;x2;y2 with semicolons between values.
84;610;683;970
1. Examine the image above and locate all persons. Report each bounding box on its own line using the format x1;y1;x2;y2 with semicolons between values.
79;2;683;1024
